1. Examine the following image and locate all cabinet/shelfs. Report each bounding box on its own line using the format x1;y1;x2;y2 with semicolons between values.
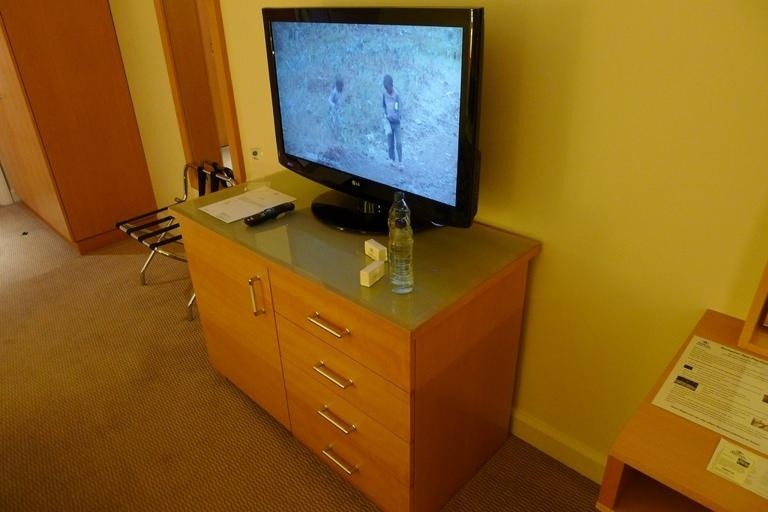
0;3;156;259
169;168;542;511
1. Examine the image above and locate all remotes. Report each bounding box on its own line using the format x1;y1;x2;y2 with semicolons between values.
244;201;296;227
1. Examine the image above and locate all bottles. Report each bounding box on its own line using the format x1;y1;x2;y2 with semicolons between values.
386;190;415;295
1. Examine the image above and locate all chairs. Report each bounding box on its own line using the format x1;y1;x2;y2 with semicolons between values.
114;163;238;319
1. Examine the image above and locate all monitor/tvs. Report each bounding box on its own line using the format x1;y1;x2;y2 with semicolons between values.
261;6;485;235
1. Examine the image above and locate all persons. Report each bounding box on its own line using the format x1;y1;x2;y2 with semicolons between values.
381;72;404;171
328;77;344;107
